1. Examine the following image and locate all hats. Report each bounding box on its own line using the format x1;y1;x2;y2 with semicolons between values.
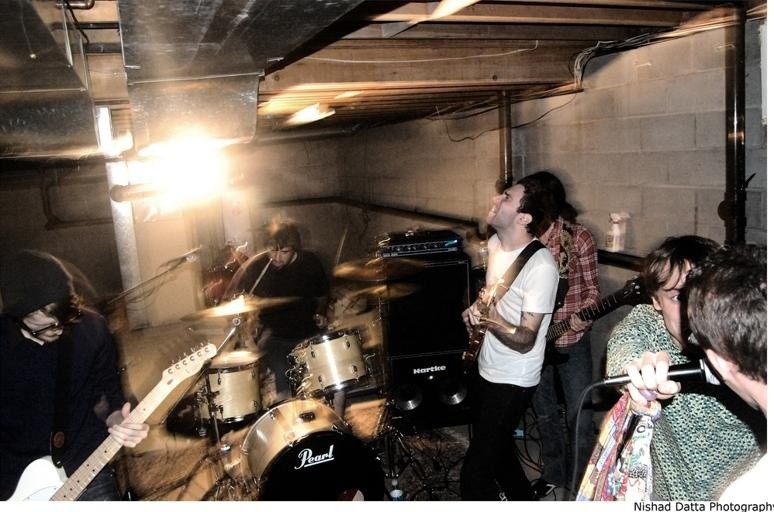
3;250;74;321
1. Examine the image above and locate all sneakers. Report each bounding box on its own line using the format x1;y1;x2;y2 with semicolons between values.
533;479;556;497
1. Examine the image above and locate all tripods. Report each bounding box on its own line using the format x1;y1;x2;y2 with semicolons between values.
372;423;430;488
176;378;241;501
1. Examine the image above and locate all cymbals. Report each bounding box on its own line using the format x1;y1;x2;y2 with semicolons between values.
179;294;299;322
370;284;415;299
331;256;428;283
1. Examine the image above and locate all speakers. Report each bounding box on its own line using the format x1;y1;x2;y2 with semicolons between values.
379;250;475;434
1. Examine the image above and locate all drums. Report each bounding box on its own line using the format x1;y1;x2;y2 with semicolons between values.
286;328;370;397
192;349;262;422
239;397;385;501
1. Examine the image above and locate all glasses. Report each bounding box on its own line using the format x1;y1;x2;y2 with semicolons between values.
21;309;82;337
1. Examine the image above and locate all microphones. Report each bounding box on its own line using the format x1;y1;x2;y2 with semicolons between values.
604;358;721;388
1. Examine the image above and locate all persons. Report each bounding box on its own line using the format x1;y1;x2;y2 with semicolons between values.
681;243;768;502
0;251;151;501
533;171;595;489
237;220;332;356
457;175;562;497
606;237;766;502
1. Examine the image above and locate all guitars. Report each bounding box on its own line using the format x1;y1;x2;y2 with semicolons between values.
545;279;641;344
460;275;504;375
2;338;218;502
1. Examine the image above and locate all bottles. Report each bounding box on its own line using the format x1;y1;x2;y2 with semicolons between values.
478;241;489;272
604;211;628;254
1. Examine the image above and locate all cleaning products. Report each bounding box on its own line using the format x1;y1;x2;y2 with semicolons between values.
604;213;623;253
